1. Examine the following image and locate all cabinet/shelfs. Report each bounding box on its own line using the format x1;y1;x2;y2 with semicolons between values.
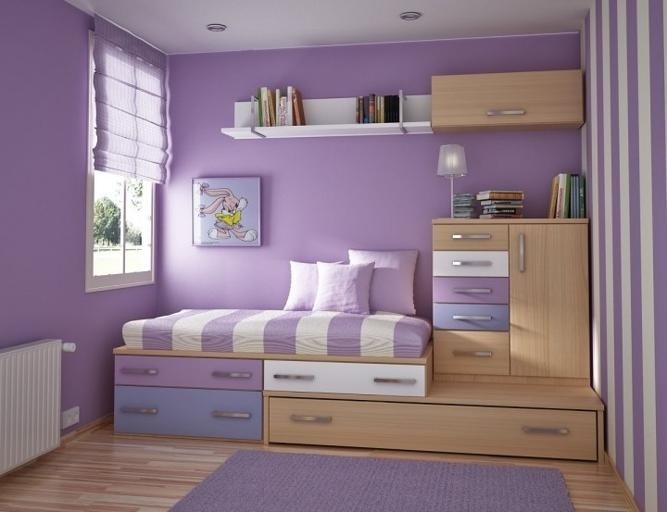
433;215;589;377
264;382;605;466
114;354;264;444
263;358;432;397
431;69;584;133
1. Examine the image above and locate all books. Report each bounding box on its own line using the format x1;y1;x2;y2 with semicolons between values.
452;189;526;220
546;173;586;219
356;88;404;124
249;84;306;127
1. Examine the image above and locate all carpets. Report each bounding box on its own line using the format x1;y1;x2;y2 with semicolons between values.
169;447;575;512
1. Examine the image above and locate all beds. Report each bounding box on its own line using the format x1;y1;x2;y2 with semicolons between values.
113;308;432;361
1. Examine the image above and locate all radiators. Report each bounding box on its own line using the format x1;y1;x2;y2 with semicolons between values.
0;339;64;476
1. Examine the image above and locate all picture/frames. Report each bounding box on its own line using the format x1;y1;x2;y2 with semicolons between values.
192;176;261;247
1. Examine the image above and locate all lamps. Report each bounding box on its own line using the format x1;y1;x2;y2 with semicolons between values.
437;144;468;218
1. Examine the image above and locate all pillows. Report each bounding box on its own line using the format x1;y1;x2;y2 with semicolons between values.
285;250;419;316
